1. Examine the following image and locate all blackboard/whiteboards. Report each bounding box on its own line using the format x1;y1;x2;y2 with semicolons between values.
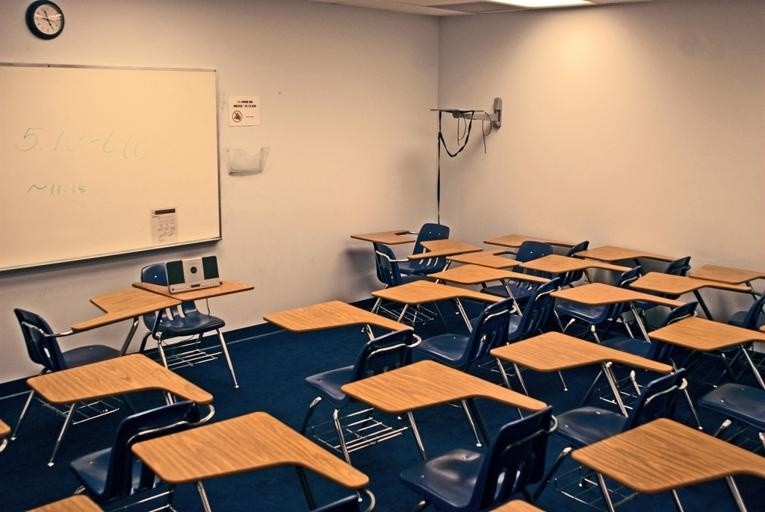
0;62;221;271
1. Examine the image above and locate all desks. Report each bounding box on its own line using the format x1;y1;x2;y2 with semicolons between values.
26;353;214;406
484;234;580;248
132;411;370;489
447;247;523;270
132;278;256;303
629;271;753;297
689;265;765;286
647;317;765;353
371;280;506;305
70;289;182;333
550;282;686;307
0;420;12;453
351;229;419;245
486;500;546;512
571;418;765;494
263;300;414;333
24;494;103;512
519;254;633;274
427;264;551;286
341;359;548;415
490;331;673;374
407;238;483;260
574;246;680;263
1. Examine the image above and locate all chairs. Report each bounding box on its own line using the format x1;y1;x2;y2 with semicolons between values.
140;263;240;403
579;301;703;430
480;241;552;314
361;242;450;332
697;382;765;449
708;293;765;390
10;308;137;468
532;366;686;512
300;328;428;466
310;495;361;512
469;277;568;392
626;256;691;337
398;223;450;275
560;265;642;389
398;405;554;512
553;240;590;287
412;298;524;448
70;400;199;512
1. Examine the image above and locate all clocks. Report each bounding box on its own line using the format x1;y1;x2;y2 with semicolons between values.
26;0;64;41
